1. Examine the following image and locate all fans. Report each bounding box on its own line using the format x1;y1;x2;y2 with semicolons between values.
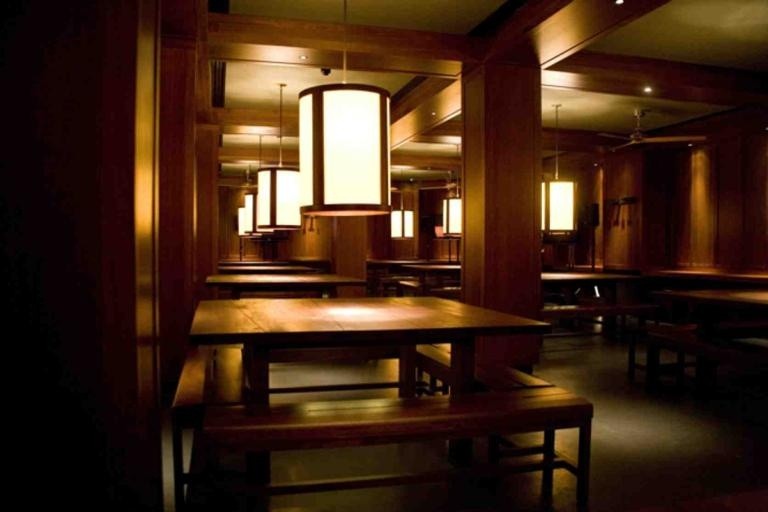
597;111;707;151
